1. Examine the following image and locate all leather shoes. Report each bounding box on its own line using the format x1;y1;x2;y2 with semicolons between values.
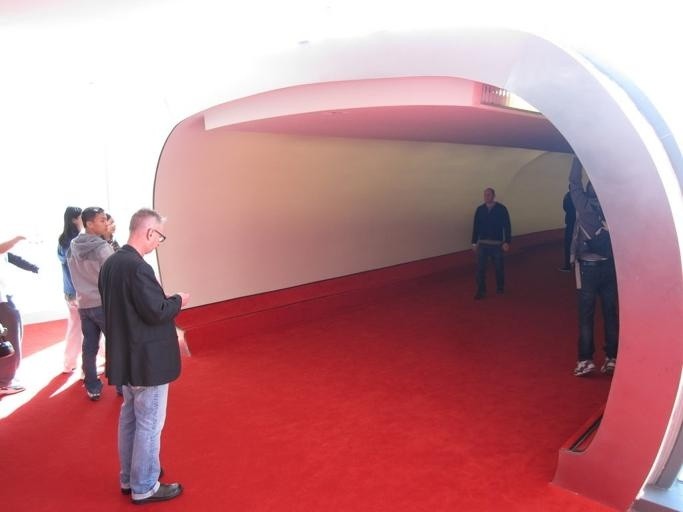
121;469;181;504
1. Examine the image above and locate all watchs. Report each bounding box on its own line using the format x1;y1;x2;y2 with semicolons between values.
471;243;476;247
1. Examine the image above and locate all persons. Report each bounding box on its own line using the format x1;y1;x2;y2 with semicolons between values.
470;187;512;300
96;206;192;505
63;205;124;402
97;211;121;254
561;154;619;379
55;205;83;375
0;234;38;401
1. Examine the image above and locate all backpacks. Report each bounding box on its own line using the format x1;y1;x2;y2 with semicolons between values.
581;212;611;256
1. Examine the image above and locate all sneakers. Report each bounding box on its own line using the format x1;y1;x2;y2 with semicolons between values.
599;358;615;374
570;360;596;377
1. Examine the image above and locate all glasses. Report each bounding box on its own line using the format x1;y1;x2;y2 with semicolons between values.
154;229;165;242
